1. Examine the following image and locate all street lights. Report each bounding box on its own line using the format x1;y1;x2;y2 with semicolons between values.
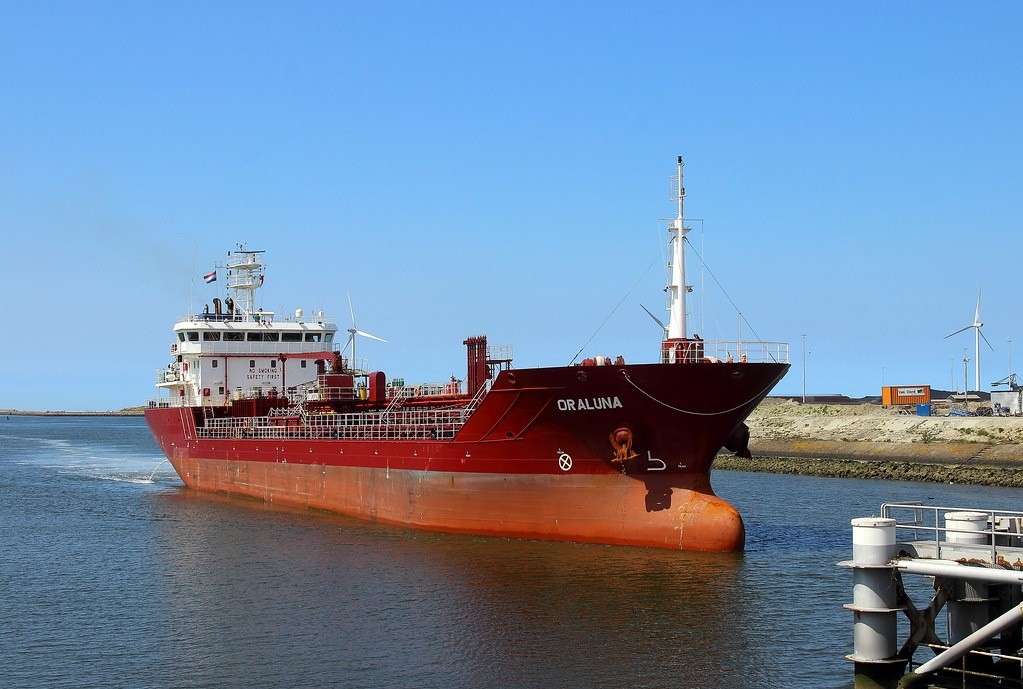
963;357;970;405
882;367;885;387
950;359;954;392
1006;340;1013;391
801;334;807;404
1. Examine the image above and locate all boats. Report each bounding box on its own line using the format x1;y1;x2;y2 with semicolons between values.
143;154;793;557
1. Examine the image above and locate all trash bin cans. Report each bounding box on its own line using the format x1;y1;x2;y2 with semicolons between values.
916;404;930;416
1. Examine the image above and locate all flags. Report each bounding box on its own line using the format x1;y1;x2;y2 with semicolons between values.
204;270;216;284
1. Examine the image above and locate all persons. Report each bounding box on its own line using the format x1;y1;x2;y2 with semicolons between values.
741;354;746;363
